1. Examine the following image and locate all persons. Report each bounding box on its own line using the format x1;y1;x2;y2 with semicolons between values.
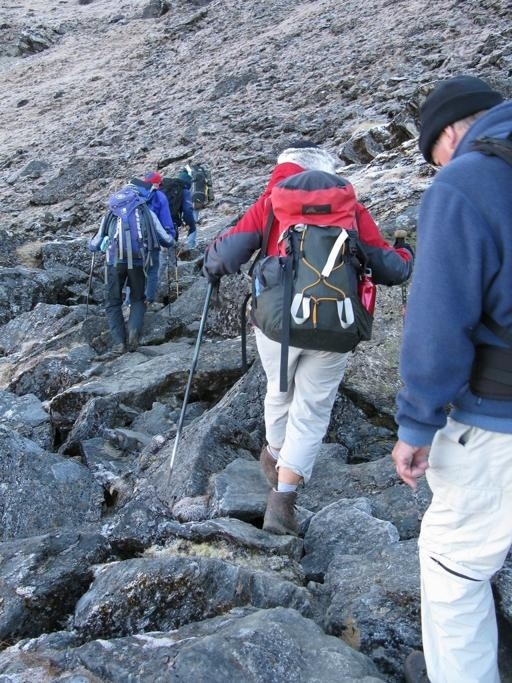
392;75;512;681
202;141;414;536
87;161;214;354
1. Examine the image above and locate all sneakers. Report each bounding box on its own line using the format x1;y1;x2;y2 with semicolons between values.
126;339;138;351
110;343;124;354
261;446;280;484
263;488;297;536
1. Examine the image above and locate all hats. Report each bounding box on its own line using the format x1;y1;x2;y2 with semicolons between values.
145;171;160;183
178;170;193;182
418;74;505;166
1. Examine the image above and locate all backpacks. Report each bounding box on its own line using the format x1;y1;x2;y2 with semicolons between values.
159;177;183;220
252;171;374;353
105;188;160;266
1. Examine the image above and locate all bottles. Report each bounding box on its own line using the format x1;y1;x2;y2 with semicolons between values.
360;268;378;315
